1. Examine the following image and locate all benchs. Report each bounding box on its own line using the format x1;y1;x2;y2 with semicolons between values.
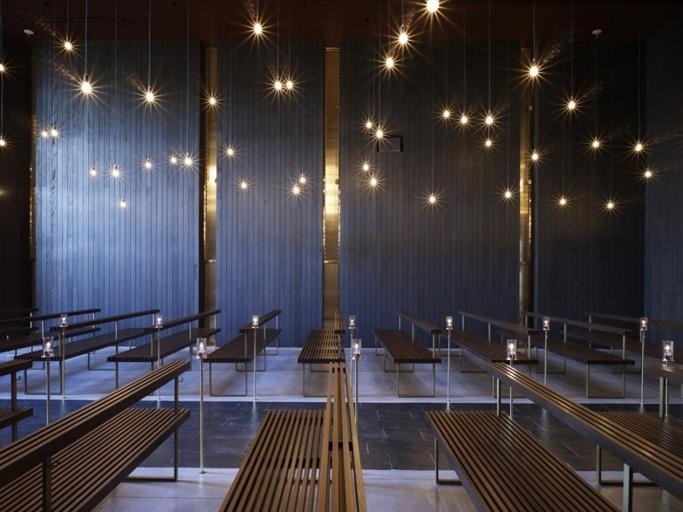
371;309;442;398
299;308;347;397
1;359;191;512
443;308;683;418
424;363;658;512
596;364;683;485
220;362;368;512
204;310;281;398
0;296;221;442
